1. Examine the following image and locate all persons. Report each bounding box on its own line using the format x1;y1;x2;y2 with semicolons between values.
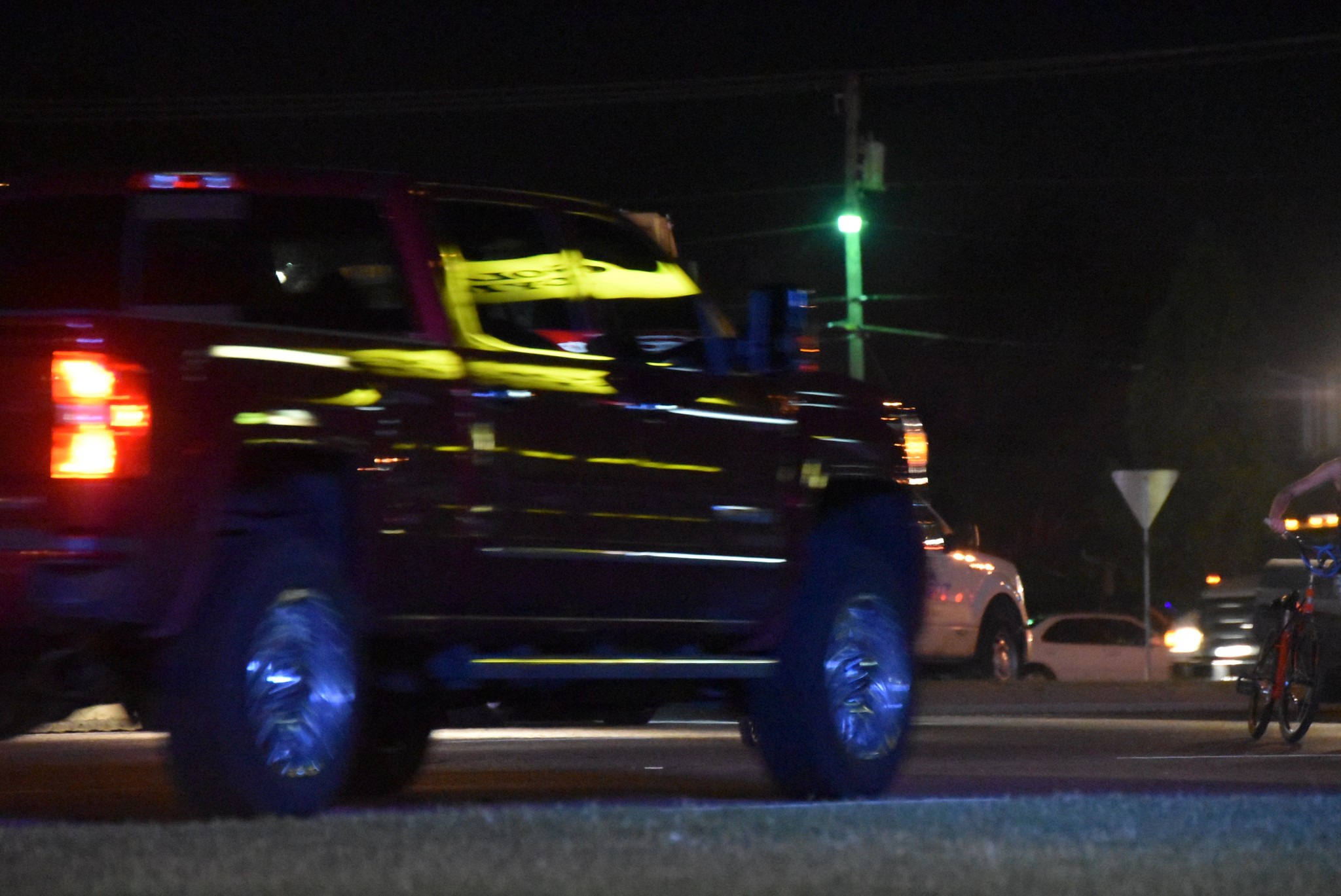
1269;457;1341;534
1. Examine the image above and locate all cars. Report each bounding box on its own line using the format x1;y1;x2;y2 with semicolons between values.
910;489;1032;682
1025;612;1173;682
1164;518;1340;697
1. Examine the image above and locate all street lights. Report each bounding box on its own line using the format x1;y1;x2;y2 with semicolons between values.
828;199;867;381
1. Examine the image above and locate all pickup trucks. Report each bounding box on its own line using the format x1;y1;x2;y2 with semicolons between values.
1;156;930;813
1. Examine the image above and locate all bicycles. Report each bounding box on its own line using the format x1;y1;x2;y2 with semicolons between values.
1232;530;1340;744
